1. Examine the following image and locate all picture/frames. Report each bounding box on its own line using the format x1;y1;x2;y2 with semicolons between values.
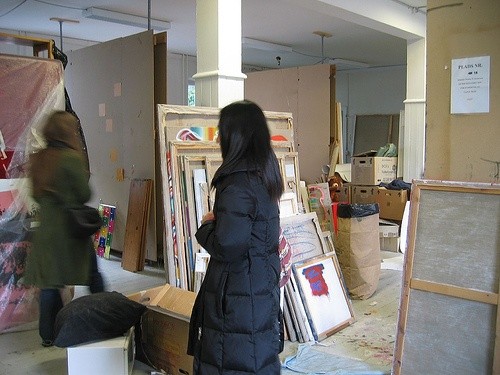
158;102;357;344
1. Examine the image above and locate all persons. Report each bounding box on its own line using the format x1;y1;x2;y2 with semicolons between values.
187;99;284;375
26;110;104;347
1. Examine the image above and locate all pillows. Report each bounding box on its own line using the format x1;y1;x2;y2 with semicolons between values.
56;290;147;347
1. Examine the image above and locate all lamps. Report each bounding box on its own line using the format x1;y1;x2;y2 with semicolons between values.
331;58;372;69
82;8;171;31
242;38;292;54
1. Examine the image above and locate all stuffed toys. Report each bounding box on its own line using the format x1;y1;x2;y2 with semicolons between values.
329;177;341;190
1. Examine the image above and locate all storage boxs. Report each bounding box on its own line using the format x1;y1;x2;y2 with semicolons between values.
331;184;408;253
127;286;198;375
67;326;136;375
351;156;397;186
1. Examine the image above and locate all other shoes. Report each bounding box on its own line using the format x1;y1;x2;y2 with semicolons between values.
41;340;53;346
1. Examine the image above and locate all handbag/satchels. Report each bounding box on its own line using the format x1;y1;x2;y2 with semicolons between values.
278;226;293;287
69;206;103;238
53;290;149;347
